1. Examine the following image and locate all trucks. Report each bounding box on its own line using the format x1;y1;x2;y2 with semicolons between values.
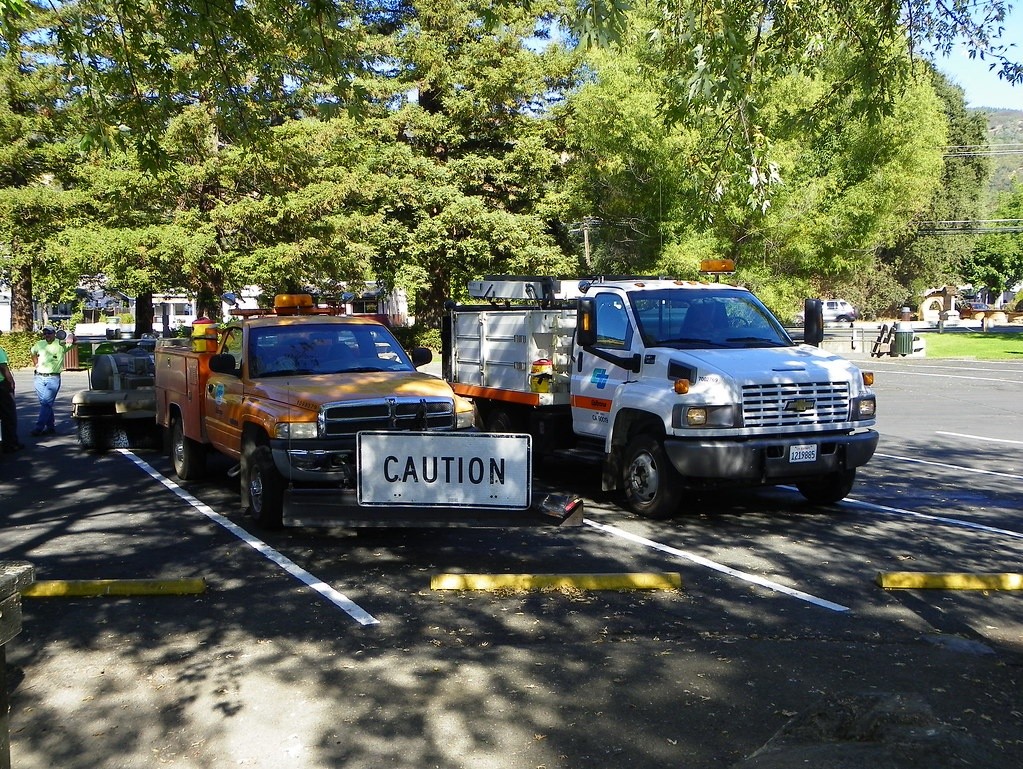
443;258;879;516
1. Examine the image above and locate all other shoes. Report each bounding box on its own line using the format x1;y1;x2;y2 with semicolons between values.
30;430;43;436
2;442;25;452
42;428;55;434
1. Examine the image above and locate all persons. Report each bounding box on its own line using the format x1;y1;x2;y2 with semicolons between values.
0;330;25;451
30;324;78;437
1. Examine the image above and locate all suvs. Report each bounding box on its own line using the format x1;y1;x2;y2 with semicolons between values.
797;300;859;324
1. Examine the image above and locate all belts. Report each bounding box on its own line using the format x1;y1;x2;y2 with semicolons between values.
36;371;60;376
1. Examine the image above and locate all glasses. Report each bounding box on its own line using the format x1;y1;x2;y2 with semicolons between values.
44;331;54;335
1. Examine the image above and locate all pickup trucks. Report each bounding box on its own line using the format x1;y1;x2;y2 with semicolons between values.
154;293;476;530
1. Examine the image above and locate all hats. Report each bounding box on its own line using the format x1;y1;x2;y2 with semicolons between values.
43;325;55;331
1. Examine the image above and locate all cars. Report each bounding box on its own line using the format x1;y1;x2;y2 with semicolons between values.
959;303;991;319
